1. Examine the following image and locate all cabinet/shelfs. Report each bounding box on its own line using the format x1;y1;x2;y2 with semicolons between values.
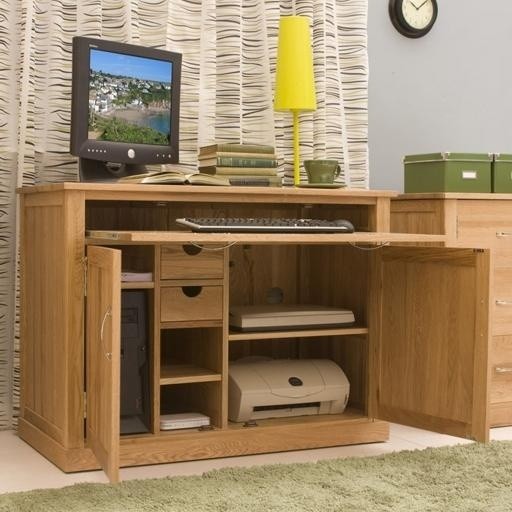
14;181;491;486
391;191;512;429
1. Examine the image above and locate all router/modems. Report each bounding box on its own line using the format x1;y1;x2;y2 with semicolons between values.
159;411;210;430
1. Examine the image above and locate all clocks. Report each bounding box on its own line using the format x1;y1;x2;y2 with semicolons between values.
389;0;438;38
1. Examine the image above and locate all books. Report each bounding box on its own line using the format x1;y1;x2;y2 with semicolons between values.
199;165;277;177
199;157;276;168
199;144;278;154
219;175;282;187
198;151;277;160
115;168;232;187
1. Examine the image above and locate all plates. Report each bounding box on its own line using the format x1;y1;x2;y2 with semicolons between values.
294;182;351;188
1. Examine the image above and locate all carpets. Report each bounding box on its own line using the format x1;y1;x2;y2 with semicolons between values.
0;440;512;512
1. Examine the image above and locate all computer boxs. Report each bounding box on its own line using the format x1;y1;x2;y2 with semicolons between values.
122;291;151;436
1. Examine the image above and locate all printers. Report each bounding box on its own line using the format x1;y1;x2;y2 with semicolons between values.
227;357;350;422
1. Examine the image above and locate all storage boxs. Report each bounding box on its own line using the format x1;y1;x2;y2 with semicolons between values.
404;152;493;192
493;153;512;193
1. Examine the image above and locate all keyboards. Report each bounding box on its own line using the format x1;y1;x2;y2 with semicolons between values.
176;217;349;233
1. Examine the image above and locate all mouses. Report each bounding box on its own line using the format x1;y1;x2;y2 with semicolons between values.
332;220;354;233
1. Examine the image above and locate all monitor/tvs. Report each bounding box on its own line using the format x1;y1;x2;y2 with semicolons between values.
70;36;182;182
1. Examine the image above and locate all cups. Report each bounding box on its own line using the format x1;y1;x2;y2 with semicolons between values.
305;160;341;184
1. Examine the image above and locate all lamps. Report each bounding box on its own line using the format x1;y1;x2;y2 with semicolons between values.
273;15;318;186
